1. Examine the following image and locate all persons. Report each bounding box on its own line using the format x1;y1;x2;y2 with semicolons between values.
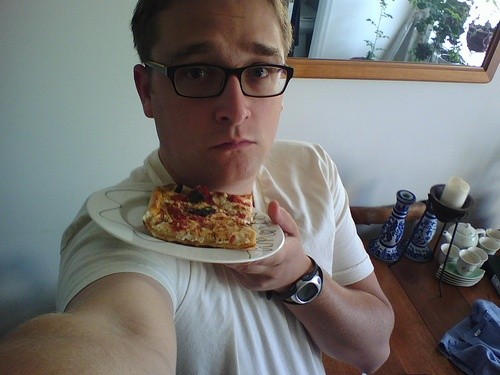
0;0;395;375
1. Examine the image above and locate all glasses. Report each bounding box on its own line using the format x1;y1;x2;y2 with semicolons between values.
141;61;295;98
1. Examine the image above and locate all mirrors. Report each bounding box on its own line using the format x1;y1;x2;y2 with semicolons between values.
285;0;500;83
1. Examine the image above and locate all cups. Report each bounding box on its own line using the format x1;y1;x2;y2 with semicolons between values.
486;226;500;240
478;236;500;256
468;246;488;268
438;243;460;263
456;249;481;277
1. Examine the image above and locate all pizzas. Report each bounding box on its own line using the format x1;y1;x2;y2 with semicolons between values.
143;185;257;249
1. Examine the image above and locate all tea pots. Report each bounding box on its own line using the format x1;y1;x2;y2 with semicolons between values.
442;222;487;251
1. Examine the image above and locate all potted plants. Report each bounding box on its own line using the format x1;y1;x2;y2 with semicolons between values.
349;0;393;61
466;22;494;52
437;41;462;65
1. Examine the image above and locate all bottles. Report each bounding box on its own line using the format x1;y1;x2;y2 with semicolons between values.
404;202;438;263
369;189;416;264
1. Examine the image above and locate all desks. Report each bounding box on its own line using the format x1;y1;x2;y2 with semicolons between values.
323;231;500;375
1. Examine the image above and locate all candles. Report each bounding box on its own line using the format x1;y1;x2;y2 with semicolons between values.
441;176;470;208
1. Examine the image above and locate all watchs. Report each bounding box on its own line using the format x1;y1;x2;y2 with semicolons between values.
266;254;324;305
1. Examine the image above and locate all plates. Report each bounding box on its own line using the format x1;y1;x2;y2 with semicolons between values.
86;184;286;264
436;262;485;287
